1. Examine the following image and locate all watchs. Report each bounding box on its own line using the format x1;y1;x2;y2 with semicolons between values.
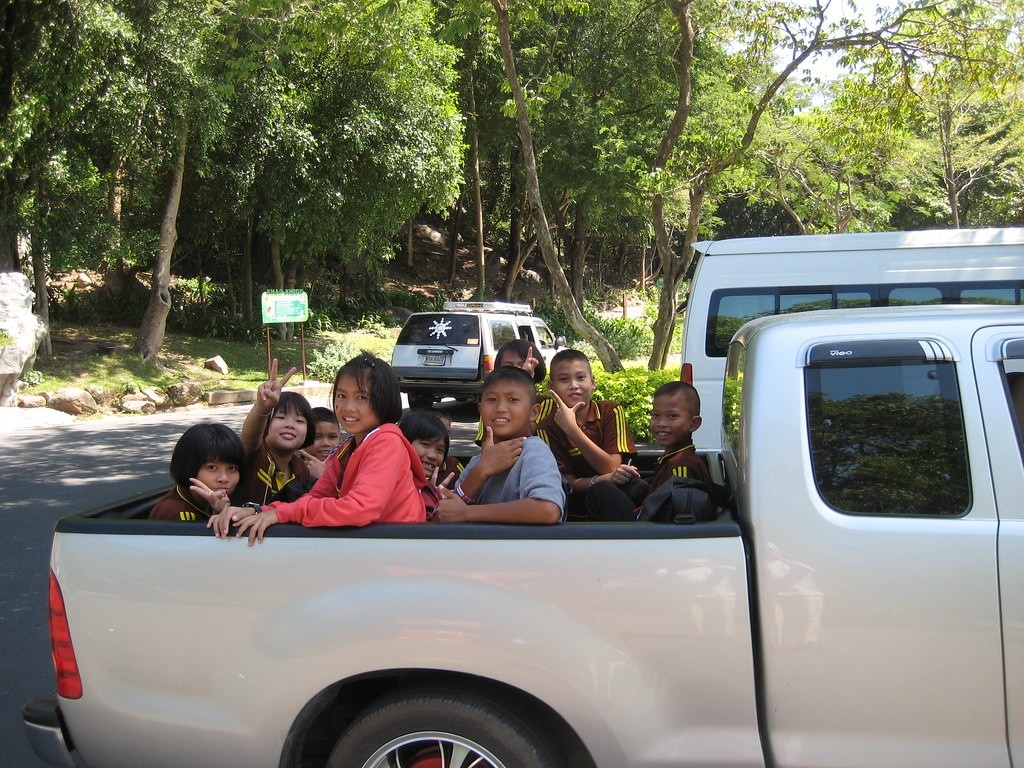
243;502;263;514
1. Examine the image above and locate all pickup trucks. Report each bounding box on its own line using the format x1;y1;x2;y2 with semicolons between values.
23;304;1024;768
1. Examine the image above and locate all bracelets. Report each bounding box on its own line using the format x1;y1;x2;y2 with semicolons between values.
456;483;475;505
589;474;600;487
252;403;275;419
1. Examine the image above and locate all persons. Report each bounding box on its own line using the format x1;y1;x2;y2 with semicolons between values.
433;367;566;527
475;340;560;451
581;380;713;522
205;351;425;548
537;347;642;492
144;359;465;525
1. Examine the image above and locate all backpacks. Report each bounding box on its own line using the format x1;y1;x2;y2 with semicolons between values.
636;475;731;523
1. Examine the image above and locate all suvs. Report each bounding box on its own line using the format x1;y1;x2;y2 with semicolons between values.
391;301;569;414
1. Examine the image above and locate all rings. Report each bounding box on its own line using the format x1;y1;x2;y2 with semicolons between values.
625;472;629;476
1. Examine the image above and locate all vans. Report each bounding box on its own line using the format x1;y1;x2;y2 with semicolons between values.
681;227;1024;456
